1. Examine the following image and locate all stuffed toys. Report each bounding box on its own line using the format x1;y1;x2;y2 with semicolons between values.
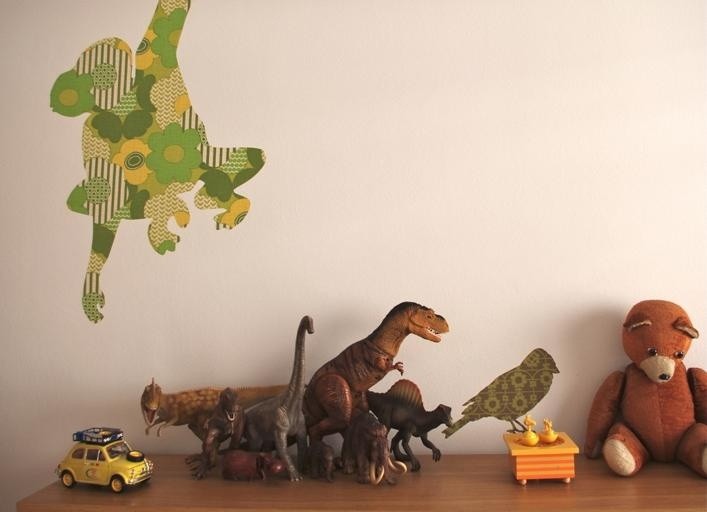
581;298;705;477
